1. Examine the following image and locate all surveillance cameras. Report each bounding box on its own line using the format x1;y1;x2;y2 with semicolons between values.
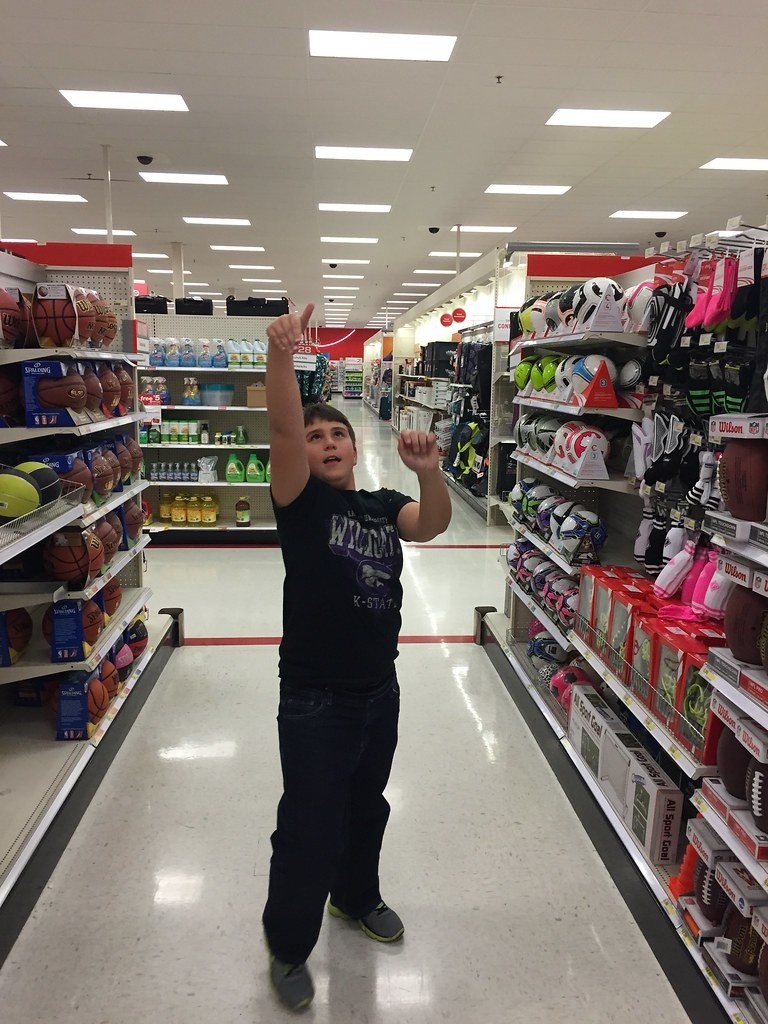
328;299;334;303
137;156;153;165
330;263;337;268
429;227;440;233
655;232;667;238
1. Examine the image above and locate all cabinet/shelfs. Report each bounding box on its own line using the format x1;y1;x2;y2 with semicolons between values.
1;243;184;968
136;313;280;545
475;250;767;1024
330;246;516;526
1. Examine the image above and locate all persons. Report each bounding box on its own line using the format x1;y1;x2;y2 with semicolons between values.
261;305;452;1011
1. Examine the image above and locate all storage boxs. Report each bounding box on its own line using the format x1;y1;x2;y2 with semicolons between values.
566;680;685;866
199;384;235;405
247;385;269;408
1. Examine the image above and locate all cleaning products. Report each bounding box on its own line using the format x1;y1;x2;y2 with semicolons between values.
136;337;274;484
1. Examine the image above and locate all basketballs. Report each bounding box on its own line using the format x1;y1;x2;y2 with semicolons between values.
2;284;146;735
691;438;768;1008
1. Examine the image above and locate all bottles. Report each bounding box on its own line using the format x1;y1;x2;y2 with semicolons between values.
235;497;250;527
226;337;267;368
171;491;219;527
159;493;173;523
142;499;153;526
266;457;271;482
226;454;244;482
246;454;265;482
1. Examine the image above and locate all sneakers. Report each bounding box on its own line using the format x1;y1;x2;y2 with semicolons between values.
263;930;314;1011
327;895;404;941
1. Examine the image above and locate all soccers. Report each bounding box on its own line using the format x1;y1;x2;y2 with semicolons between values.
504;273;661;714
